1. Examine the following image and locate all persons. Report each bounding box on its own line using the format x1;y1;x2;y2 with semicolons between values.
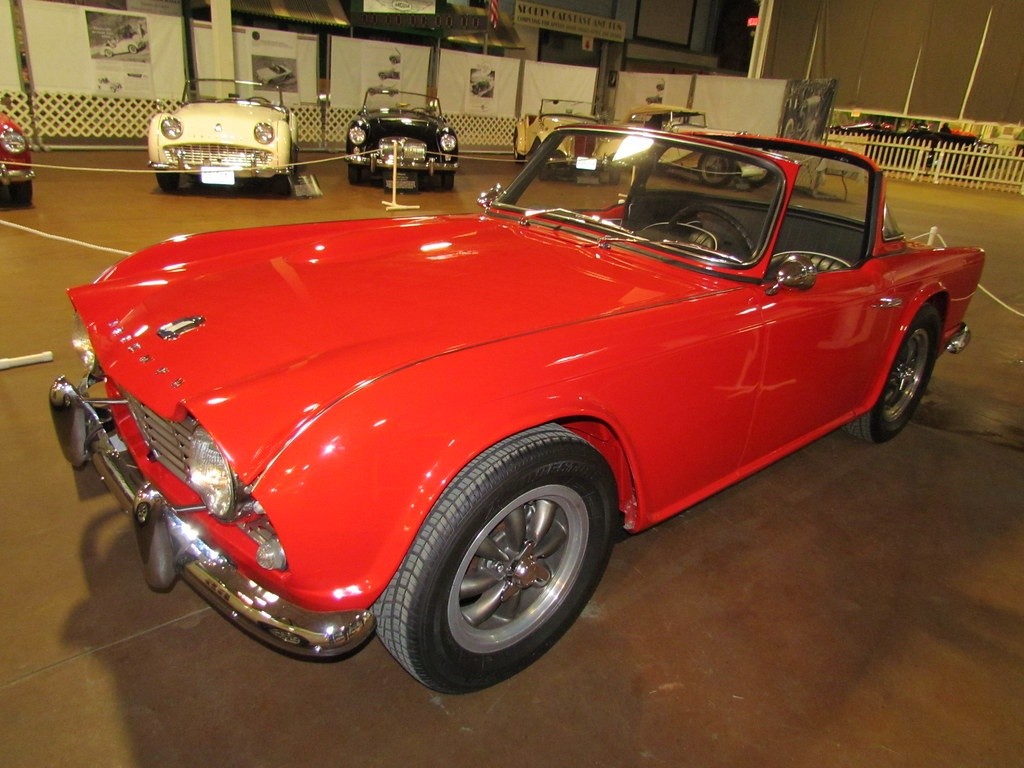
940;122;950;133
914;119;927;129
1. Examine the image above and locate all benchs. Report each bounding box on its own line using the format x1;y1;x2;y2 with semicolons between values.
625;191;866;267
527;115;537;125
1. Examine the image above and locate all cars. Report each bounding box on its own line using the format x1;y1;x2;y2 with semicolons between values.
513;98;777;189
344;88;461;190
147;78;300;197
48;122;986;696
829;122;1024;182
256;64;293;87
0;111;36;208
99;24;148;58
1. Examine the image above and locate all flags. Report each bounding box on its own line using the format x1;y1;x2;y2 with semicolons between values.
490;0;499;29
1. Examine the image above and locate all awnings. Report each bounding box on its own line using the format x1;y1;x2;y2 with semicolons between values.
206;0;349;28
